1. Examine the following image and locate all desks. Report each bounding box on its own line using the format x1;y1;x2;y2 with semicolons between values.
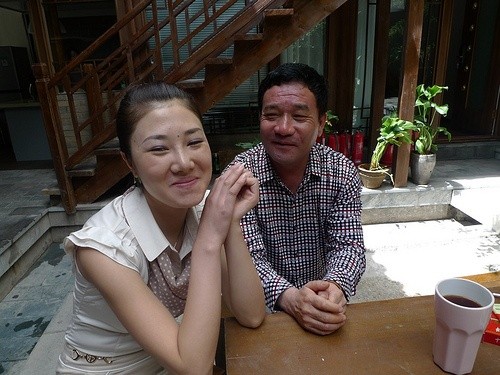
0;98;53;161
224;288;500;375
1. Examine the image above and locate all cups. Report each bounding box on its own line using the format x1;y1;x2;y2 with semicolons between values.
433;278;495;374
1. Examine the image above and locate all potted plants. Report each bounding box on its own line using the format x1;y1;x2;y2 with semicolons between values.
357;106;421;189
414;83;452;185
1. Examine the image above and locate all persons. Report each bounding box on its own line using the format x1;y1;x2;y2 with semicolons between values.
54;81;265;375
222;63;368;337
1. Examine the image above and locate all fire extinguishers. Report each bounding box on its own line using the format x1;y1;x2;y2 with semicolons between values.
316;125;363;168
382;128;420;167
